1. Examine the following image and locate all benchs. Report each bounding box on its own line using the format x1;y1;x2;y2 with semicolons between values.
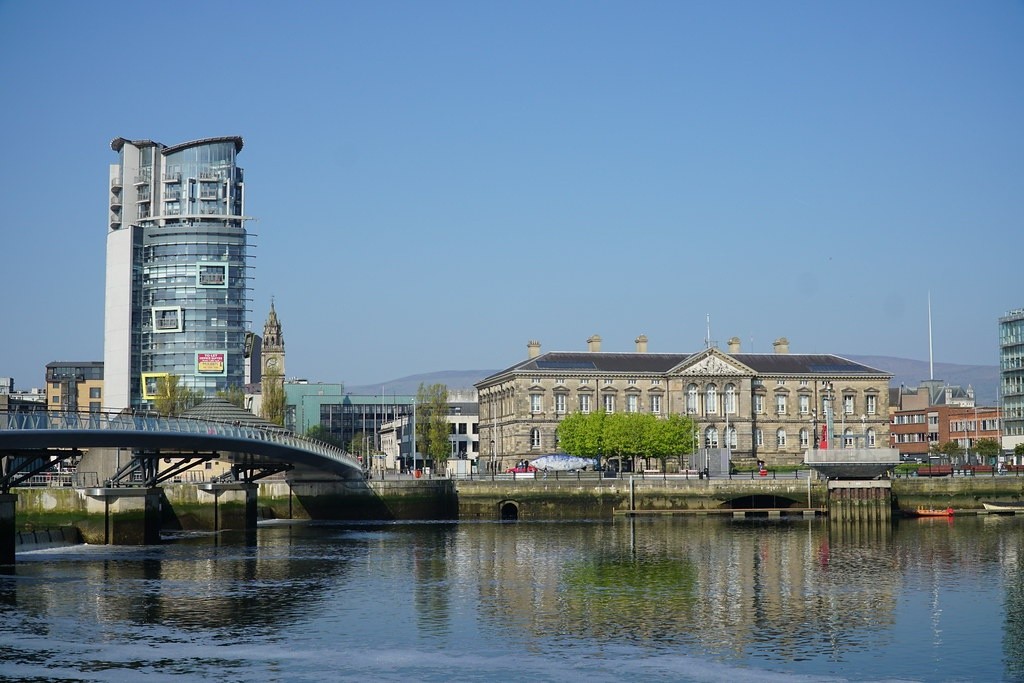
643;469;660;474
679;470;697;474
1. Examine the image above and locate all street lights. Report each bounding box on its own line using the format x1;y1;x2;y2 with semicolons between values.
927;435;932;478
992;400;1000;461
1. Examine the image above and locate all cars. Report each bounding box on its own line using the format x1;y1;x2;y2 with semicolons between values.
505;465;538;473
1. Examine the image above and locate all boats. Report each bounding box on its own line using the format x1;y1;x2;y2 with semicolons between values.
982;501;1024;514
903;507;978;516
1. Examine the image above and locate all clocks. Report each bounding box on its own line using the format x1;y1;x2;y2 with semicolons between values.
266;357;278;370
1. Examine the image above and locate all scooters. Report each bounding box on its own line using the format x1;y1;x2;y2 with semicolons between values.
997;461;1008;475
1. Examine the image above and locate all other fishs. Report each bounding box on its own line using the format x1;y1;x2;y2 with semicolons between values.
528;453;599;471
